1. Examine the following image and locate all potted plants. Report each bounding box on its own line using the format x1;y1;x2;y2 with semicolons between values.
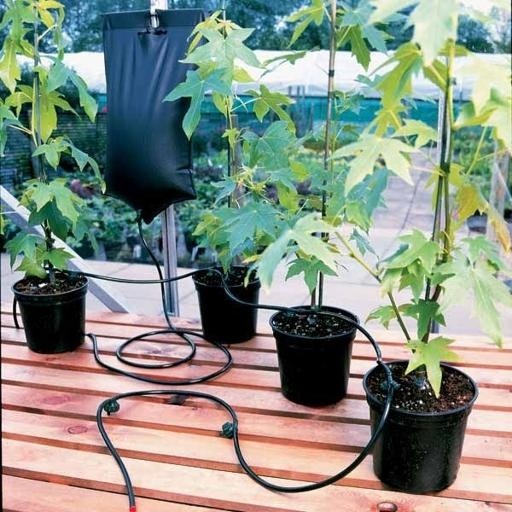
0;0;108;354
